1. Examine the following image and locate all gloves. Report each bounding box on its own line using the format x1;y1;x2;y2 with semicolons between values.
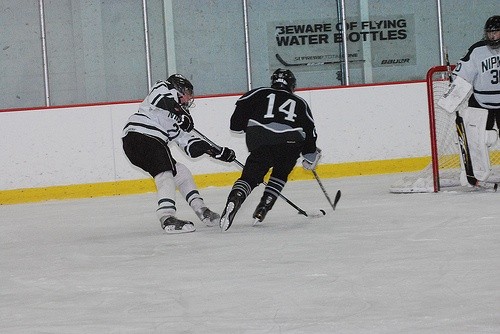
303;151;321;170
175;110;194;133
210;147;236;163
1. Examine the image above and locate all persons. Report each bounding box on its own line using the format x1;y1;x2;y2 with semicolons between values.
122;74;236;233
219;68;322;233
436;15;500;187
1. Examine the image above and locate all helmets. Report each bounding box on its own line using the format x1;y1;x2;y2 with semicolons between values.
271;68;296;94
484;16;500;47
165;73;195;108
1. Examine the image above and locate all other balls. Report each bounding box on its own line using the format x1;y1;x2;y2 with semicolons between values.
298;212;303;214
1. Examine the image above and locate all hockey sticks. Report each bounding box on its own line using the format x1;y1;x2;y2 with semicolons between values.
442;45;498;193
312;170;341;211
191;127;326;218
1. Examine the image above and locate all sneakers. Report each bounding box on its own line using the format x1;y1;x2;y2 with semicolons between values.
159;215;195;233
252;194;278;227
194;206;220;226
219;189;246;233
